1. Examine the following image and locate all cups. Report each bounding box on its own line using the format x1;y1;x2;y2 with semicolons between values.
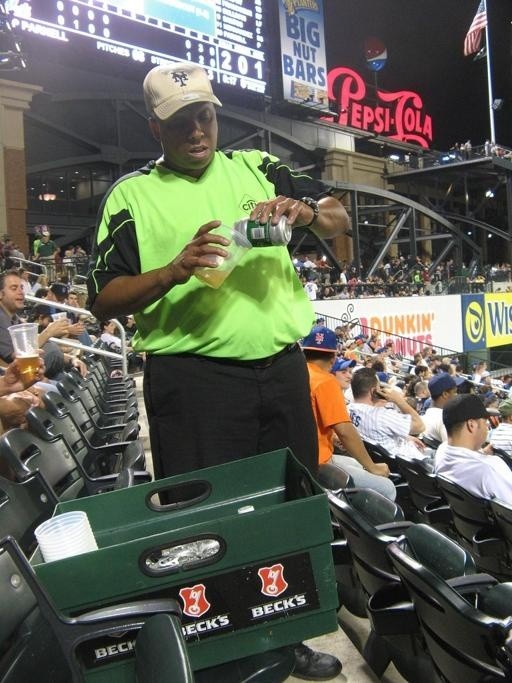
192;224;252;290
6;323;42;374
49;311;68;322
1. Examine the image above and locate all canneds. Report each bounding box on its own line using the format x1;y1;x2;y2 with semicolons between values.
233;215;292;247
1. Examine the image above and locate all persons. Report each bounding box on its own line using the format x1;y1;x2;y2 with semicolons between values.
452;138;512;161
289;252;511;301
0;224;136;441
298;318;508;510
84;59;351;504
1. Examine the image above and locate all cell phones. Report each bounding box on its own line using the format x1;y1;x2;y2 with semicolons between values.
374;392;386;399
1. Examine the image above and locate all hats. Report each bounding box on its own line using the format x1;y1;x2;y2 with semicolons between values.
50;283;70;298
144;61;222;122
300;316;511;424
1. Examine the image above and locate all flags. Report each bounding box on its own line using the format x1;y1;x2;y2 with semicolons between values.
462;0;487;57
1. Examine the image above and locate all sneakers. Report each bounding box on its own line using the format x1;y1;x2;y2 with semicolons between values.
286;643;343;680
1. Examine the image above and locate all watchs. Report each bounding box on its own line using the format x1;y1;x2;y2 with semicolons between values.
297;196;319;229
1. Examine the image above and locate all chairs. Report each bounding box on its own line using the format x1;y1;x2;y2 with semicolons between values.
0;361;512;683
